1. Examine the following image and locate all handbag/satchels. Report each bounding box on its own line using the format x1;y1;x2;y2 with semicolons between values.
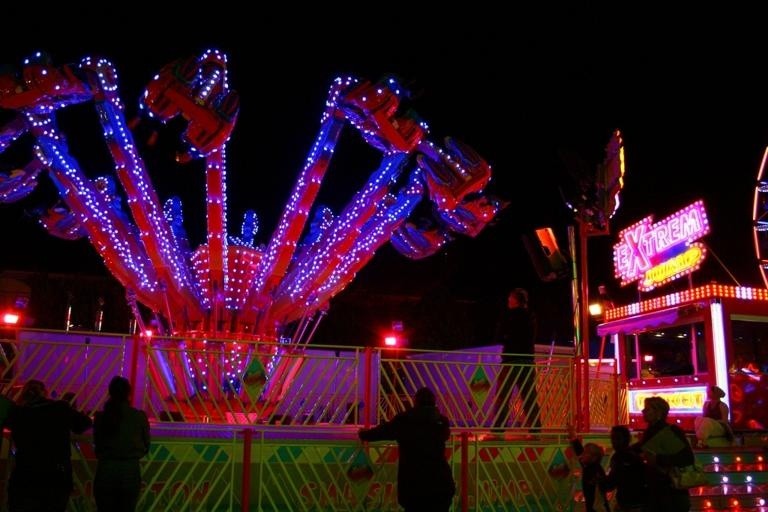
669;464;705;489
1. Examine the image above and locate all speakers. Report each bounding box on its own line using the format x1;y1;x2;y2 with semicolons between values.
523;226;567;282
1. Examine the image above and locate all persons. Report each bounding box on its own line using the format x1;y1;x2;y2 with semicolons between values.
1;390;17;457
92;374;150;510
9;379;92;511
565;419;610;510
695;386;735;448
475;286;542;439
360;386;454;512
624;396;705;511
598;425;652;511
